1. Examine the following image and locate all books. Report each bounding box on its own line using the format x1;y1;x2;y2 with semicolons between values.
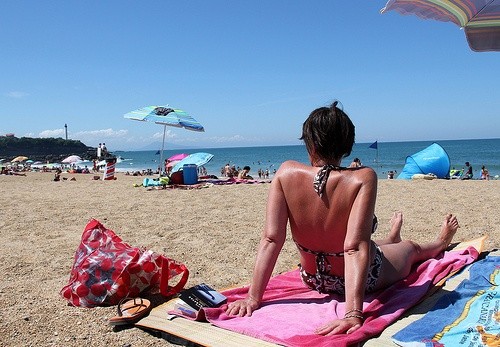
174;283;228;317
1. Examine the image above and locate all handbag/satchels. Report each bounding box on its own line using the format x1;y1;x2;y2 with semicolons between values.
59;216;189;308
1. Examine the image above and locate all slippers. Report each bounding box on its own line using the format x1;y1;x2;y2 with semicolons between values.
107;296;152;326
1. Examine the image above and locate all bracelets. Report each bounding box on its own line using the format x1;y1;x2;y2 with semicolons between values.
344;308;364;320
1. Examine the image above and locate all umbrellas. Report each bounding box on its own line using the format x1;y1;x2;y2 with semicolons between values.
169;152;215;176
380;1;500;52
166;160;182;167
167;153;191;161
0;156;43;165
122;105;206;178
61;154;82;163
97;157;134;165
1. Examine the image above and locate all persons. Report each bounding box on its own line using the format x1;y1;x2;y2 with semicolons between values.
98;143;109;157
124;158;168;176
350;157;363;168
452;161;490;180
197;160;277;184
0;162;91;175
92;160;99;173
222;99;460;337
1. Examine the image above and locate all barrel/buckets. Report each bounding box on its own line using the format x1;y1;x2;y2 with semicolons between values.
183;164;198;185
